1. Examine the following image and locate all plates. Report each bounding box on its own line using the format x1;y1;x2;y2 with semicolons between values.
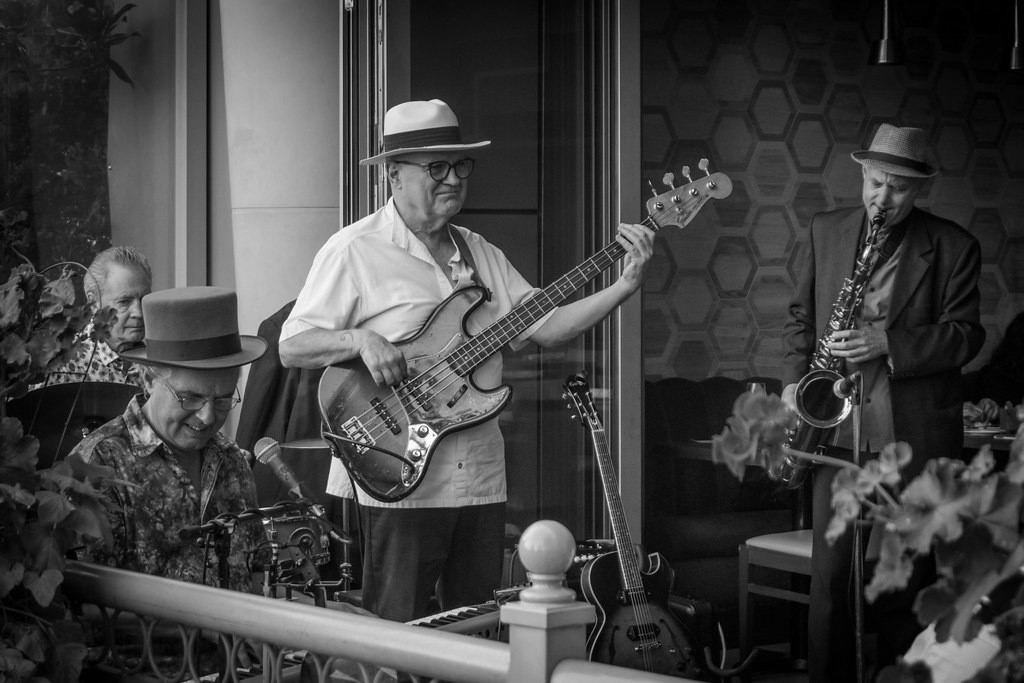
994;433;1016;441
691;439;712;446
965;426;1006;436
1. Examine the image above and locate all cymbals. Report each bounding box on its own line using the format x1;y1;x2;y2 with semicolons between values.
279;438;332;449
6;381;145;468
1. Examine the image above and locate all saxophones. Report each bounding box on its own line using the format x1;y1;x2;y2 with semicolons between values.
779;208;888;491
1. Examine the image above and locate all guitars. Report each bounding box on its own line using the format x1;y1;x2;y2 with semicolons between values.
560;373;707;680
317;159;734;503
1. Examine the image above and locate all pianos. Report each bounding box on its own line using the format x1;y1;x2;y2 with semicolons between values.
181;600;509;683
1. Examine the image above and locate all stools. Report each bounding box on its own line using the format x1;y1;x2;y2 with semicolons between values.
735;530;813;673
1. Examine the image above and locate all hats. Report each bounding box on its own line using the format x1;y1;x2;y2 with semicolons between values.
850;122;942;179
120;285;270;368
359;98;492;166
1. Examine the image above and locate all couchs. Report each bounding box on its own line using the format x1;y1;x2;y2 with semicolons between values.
643;377;818;671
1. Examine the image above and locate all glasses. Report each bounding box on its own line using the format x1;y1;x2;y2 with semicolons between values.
147;366;241;414
395;156;477;182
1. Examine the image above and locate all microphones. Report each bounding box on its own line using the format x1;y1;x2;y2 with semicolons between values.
833;371;862;399
254;438;333;534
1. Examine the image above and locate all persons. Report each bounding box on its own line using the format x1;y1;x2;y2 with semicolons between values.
35;246;154;389
280;99;656;623
782;122;986;683
45;285;380;673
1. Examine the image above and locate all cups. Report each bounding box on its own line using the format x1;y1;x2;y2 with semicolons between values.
745;382;766;396
